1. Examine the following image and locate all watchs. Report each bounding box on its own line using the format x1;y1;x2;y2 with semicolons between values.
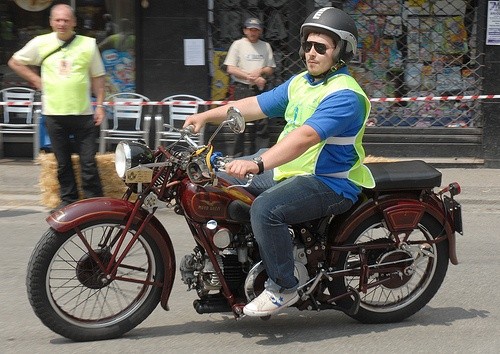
252;156;265;175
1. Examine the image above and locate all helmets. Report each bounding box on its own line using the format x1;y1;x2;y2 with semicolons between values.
299;7;358;62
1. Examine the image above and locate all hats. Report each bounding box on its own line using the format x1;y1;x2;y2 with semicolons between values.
244;18;262;31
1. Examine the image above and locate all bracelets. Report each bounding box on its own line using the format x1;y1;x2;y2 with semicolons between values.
97;104;105;108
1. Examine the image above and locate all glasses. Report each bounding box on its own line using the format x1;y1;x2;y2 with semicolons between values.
302;41;336;54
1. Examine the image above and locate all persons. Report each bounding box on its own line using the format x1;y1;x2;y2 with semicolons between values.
223;18;276;158
0;16;14;65
102;14;120;38
8;4;106;215
183;7;376;316
97;19;136;52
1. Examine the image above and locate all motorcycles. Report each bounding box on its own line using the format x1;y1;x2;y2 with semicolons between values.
26;106;463;342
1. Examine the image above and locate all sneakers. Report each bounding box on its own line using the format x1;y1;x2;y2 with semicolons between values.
243;289;300;317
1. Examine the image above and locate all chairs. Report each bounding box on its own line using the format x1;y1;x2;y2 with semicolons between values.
99;92;152;155
155;94;208;153
0;87;41;160
